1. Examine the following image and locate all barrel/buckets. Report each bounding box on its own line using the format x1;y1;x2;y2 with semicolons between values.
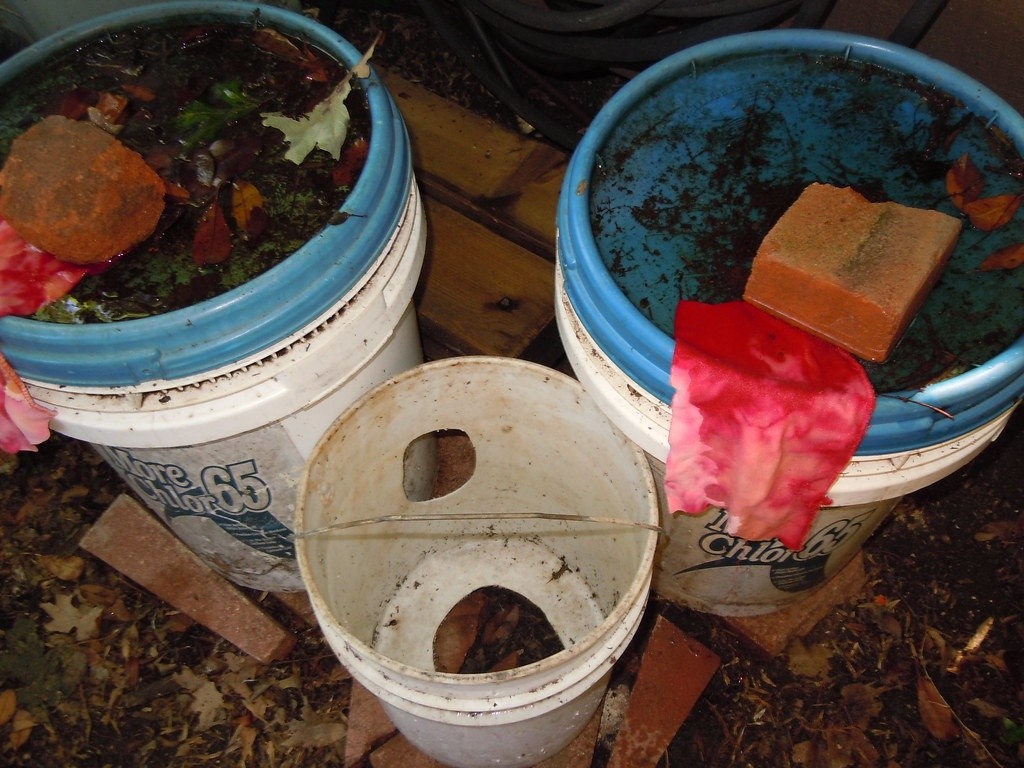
553;28;1024;617
295;354;666;768
0;0;430;594
